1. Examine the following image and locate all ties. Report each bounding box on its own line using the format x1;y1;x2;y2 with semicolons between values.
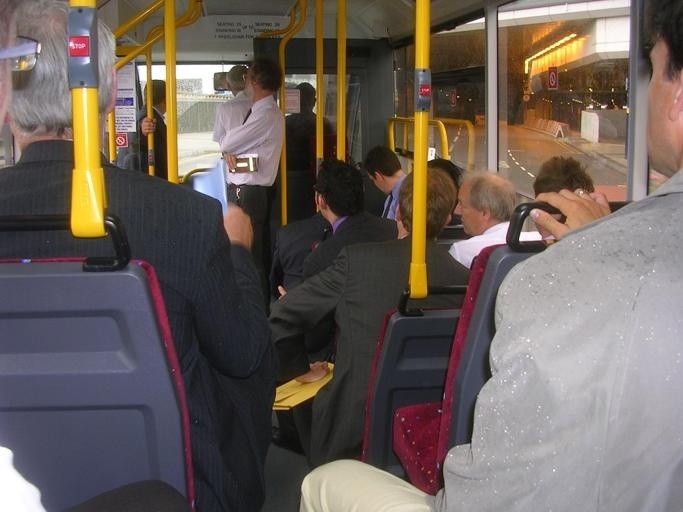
384;194;394;219
242;108;252;125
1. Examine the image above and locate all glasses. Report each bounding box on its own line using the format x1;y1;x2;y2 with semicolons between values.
0;36;41;73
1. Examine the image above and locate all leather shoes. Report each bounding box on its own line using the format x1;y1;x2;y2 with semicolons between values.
270;427;299;453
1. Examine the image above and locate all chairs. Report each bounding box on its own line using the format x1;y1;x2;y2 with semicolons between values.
0;215;195;510
362;285;464;464
394;202;630;495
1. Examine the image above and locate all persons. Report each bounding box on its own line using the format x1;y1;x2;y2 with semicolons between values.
136;80;168;181
267;167;470;473
0;2;279;509
299;0;683;510
269;210;334;301
217;75;228;90
214;66;253;208
275;81;333;222
219;57;285;264
365;145;597;270
272;159;399;457
